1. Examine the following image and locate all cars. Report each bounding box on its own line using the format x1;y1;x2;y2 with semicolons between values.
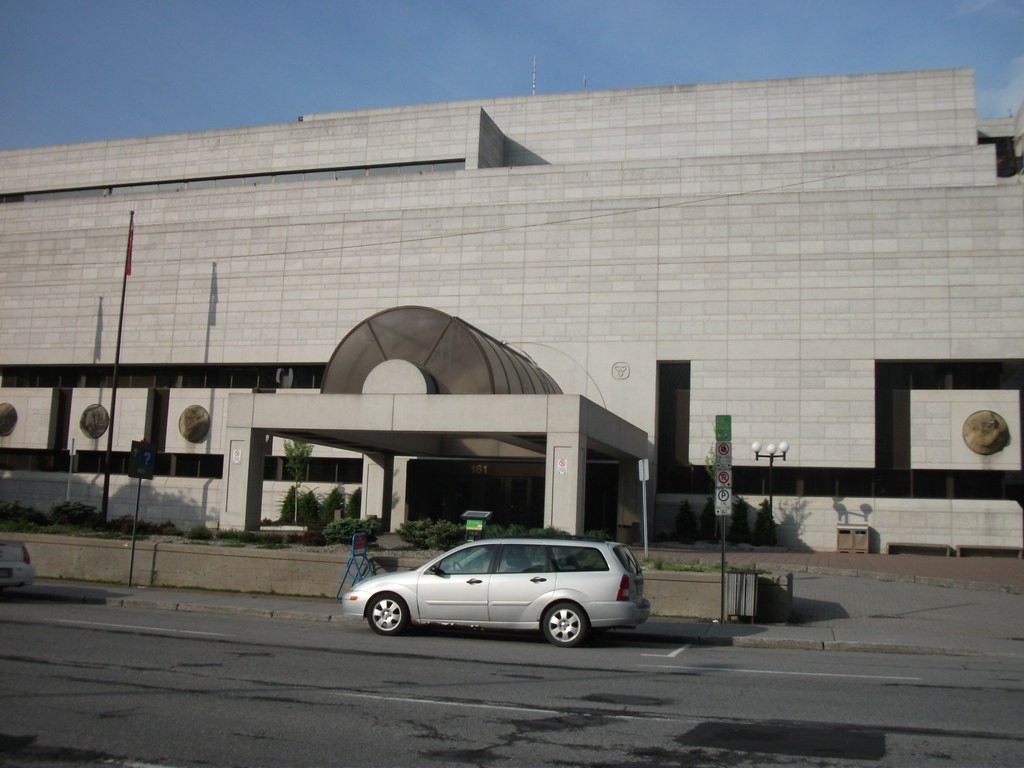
0;539;36;595
340;537;651;648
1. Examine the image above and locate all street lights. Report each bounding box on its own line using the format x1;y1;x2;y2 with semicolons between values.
751;440;790;547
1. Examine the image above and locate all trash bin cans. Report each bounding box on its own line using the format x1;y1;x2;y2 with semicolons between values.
837;524;871;554
723;572;759;624
